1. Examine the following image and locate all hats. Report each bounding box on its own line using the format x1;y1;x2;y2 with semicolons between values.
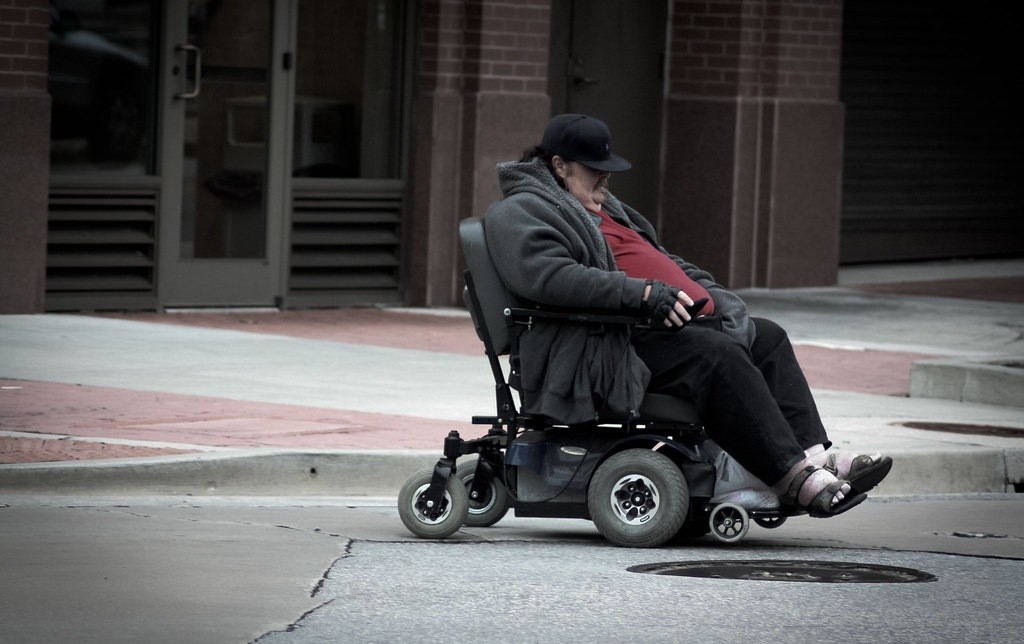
542;113;633;172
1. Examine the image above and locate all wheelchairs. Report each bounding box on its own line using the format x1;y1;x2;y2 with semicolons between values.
396;217;807;550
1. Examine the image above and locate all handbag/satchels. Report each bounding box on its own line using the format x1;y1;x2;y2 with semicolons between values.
700;438;780;510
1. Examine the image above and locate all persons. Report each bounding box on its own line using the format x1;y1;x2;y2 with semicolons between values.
483;113;892;519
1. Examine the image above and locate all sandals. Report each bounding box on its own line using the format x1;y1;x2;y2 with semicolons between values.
822;453;893;494
779;466;867;518
